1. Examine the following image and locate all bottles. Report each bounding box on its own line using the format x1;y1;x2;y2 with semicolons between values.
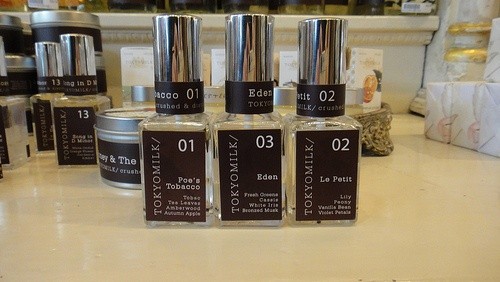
280;15;363;226
49;34;112;170
211;12;287;227
0;36;38;172
135;13;219;229
29;41;66;155
